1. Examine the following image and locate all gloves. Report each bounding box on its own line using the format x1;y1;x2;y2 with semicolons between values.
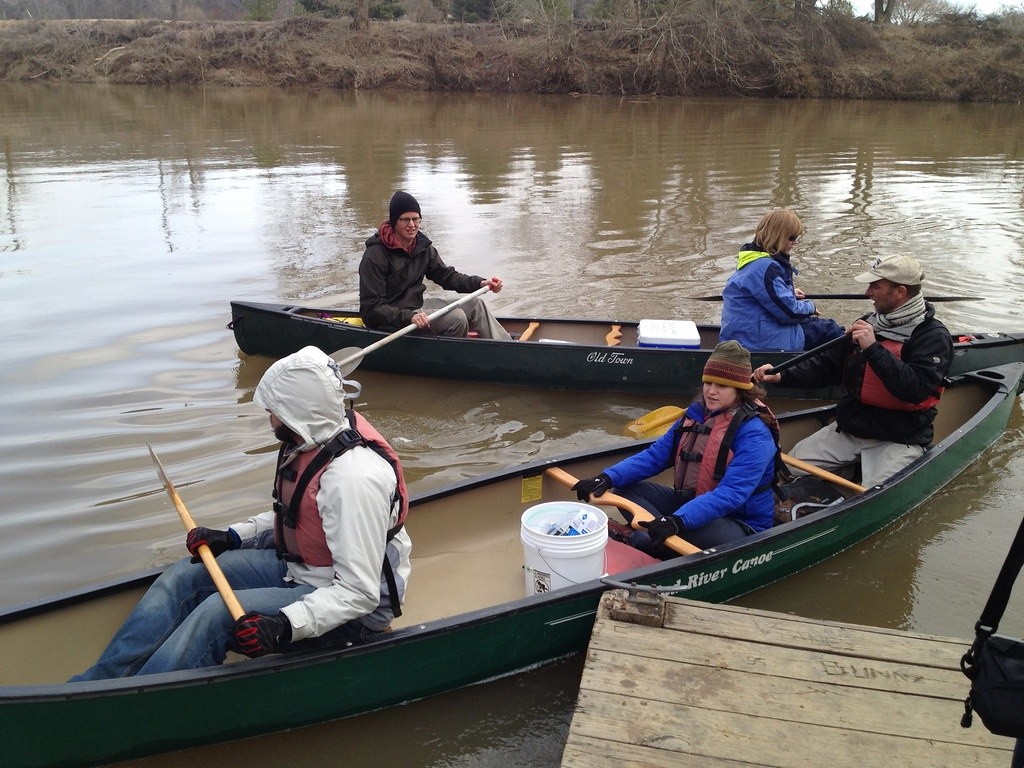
571;473;613;503
186;526;236;564
234;611;291;658
638;515;684;545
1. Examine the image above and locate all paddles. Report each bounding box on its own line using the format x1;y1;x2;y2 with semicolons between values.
677;293;987;302
622;331;854;442
326;276;498;379
143;439;246;622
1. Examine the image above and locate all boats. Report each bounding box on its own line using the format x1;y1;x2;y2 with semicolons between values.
0;359;1024;768
223;297;1024;390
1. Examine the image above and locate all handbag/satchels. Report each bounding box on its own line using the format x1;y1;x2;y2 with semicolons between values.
960;637;1024;738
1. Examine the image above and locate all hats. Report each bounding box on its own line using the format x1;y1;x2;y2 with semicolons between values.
702;340;754;389
854;254;925;285
389;191;422;226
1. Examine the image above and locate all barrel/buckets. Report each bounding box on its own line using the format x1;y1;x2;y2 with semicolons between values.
519;501;611;597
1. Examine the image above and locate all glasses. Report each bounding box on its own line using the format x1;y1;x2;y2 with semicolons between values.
789;236;798;241
398;218;419;221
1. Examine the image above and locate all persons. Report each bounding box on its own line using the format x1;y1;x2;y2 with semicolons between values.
358;190;514;341
750;254;955;494
570;339;778;561
718;207;822;352
66;344;413;684
